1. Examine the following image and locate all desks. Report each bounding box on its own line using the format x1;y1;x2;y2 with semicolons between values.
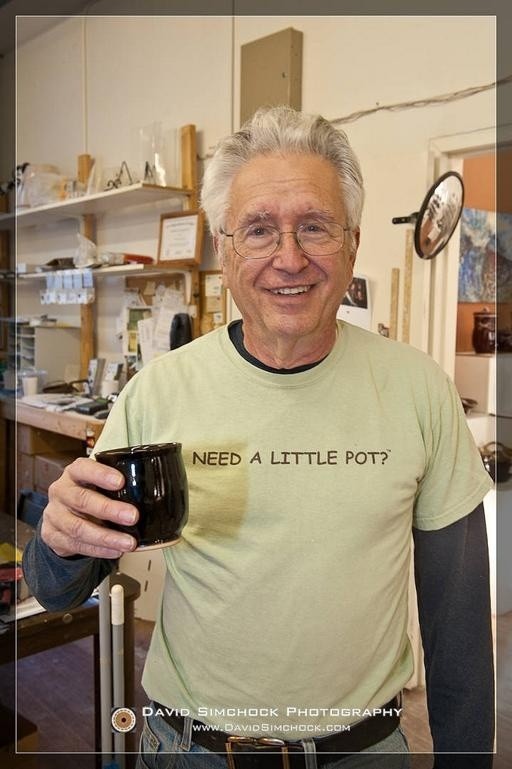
1;396;107;515
0;510;142;769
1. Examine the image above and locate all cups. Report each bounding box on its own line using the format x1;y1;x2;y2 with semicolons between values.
472;309;496;353
23;377;38;396
94;441;190;553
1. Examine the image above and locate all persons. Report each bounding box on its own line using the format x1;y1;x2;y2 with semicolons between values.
20;105;496;767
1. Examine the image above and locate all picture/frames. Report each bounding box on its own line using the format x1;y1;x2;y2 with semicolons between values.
156;209;206;267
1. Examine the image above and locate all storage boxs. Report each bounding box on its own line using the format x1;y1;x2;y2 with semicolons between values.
8;421;88;514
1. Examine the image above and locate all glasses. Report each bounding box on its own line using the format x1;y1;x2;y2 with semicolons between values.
217;220;351;258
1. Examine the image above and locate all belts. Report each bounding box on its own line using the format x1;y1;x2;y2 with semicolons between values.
153;693;399;768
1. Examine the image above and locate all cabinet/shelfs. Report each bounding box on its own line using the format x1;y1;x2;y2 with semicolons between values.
0;124;200;398
